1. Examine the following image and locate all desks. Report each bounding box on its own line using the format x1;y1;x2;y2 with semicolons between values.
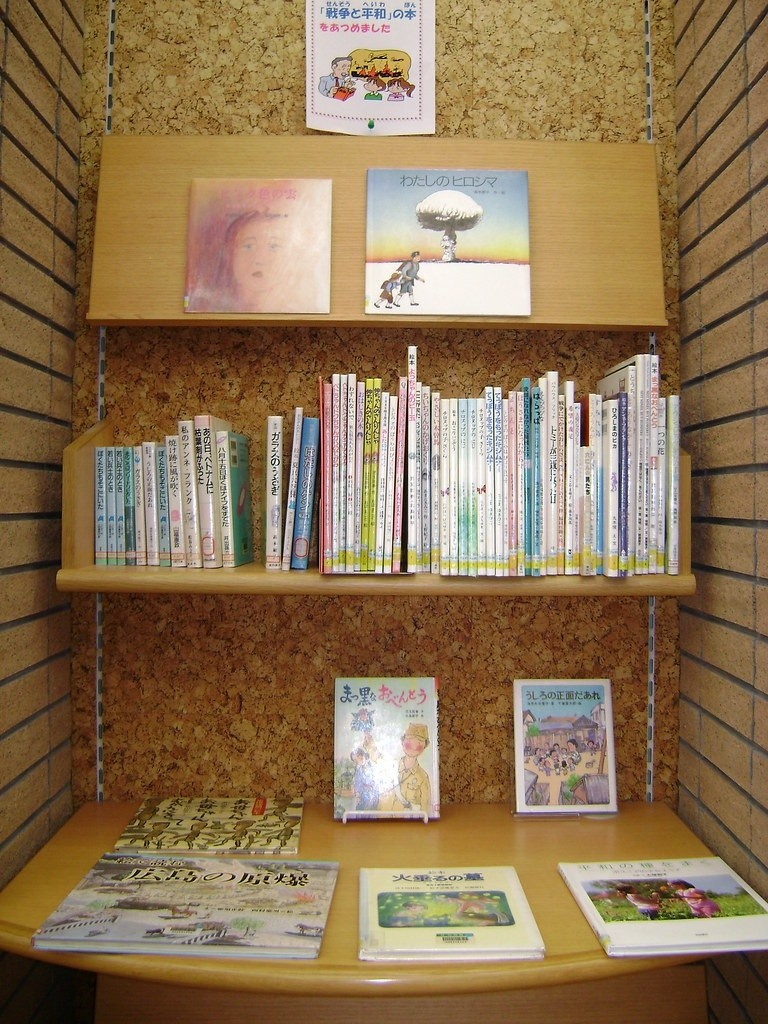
0;797;767;1024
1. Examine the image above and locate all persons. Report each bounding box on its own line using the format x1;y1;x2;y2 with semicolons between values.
616;883;662;921
667;879;720;918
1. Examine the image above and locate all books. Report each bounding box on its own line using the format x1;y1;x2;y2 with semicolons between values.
557;856;768;957
31;852;340;959
513;678;618;814
364;169;531;317
357;866;545;962
94;416;254;569
333;676;440;820
114;795;304;854
185;178;332;314
263;344;680;578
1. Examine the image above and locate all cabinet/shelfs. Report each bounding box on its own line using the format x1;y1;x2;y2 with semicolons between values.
55;308;699;598
